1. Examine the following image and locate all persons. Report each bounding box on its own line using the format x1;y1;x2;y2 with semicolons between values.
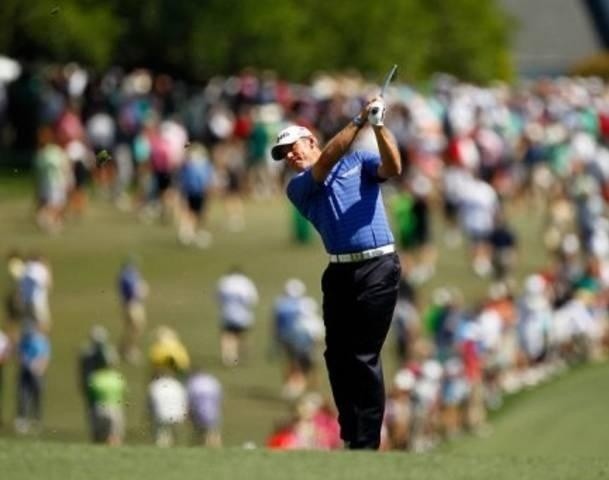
2;257;345;452
31;72;319;246
271;96;401;452
311;74;609;454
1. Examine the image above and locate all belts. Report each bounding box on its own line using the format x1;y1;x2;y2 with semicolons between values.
329;245;394;263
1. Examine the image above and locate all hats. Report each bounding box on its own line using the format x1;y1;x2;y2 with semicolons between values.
272;126;313;160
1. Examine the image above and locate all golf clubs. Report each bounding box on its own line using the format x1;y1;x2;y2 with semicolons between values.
371;64;399;115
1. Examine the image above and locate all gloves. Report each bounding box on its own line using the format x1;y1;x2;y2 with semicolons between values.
368;97;387;126
